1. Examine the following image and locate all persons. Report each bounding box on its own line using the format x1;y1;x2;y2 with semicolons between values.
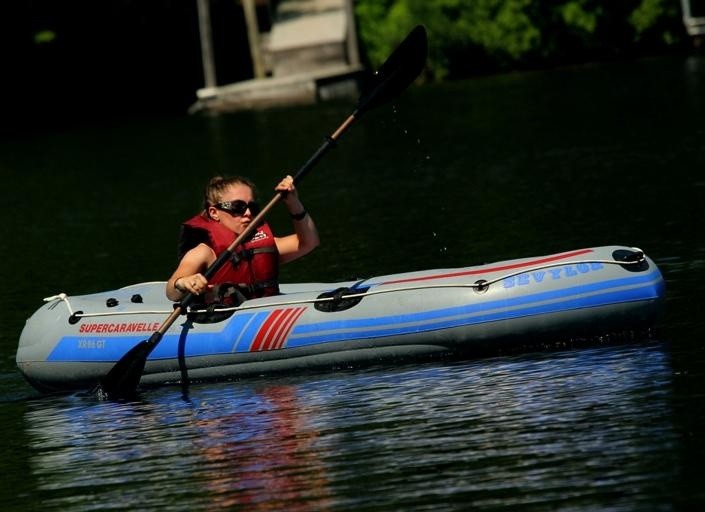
163;167;324;313
188;384;332;512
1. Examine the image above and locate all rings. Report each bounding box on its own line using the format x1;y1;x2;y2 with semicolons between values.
191;282;196;288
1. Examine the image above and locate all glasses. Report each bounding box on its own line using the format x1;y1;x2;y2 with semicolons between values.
216;199;260;217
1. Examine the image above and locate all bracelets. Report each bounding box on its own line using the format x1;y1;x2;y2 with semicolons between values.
173;276;183;288
289;208;307;221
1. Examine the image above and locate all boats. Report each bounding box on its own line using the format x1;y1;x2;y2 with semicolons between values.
14;243;668;398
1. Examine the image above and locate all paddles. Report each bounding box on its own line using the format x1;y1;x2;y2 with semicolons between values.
98;24;426;396
